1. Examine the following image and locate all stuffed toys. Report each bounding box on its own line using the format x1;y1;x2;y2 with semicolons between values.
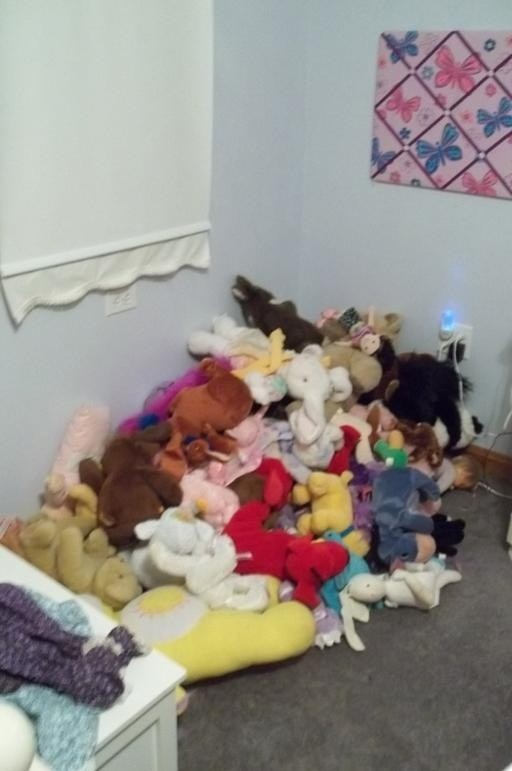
2;268;489;713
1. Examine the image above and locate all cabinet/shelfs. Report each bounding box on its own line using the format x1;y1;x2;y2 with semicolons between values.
0;543;187;771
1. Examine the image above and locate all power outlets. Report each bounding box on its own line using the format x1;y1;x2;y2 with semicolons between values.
436;324;472;358
